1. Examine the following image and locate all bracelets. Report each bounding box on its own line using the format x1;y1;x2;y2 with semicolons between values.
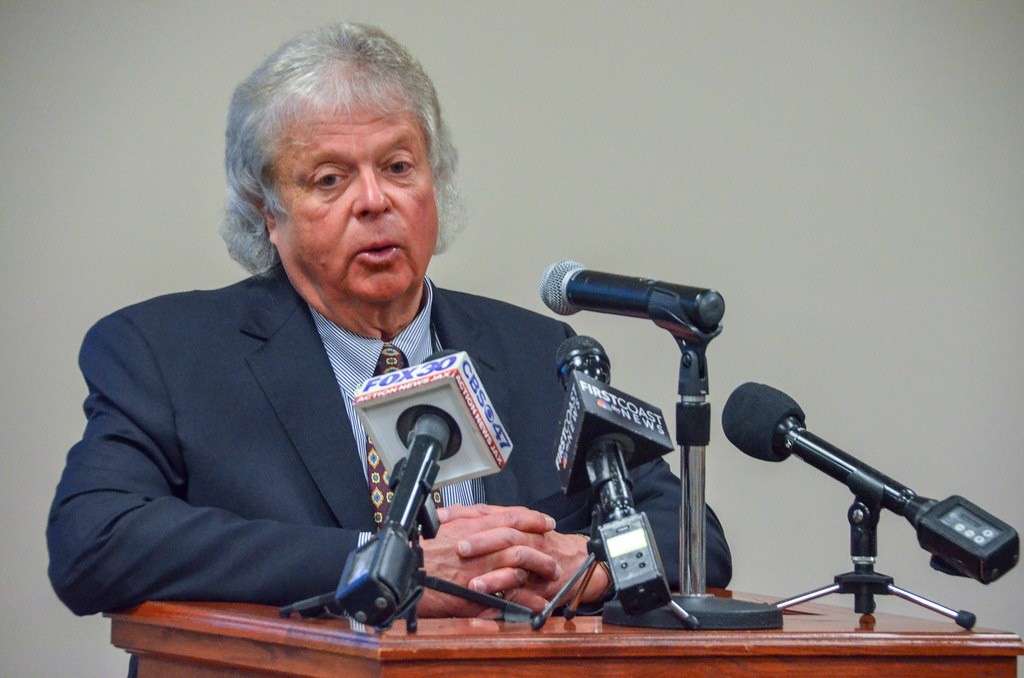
577;533;616;605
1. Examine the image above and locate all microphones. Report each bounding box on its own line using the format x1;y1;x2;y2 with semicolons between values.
350;349;535;544
554;335;673;525
538;260;726;331
722;381;918;517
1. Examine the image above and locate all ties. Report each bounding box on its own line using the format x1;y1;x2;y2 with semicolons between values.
367;342;408;528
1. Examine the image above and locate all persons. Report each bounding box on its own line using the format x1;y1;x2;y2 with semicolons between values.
46;18;732;617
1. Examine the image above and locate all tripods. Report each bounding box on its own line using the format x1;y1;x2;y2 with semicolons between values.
768;495;1019;630
531;512;703;631
278;546;539;635
